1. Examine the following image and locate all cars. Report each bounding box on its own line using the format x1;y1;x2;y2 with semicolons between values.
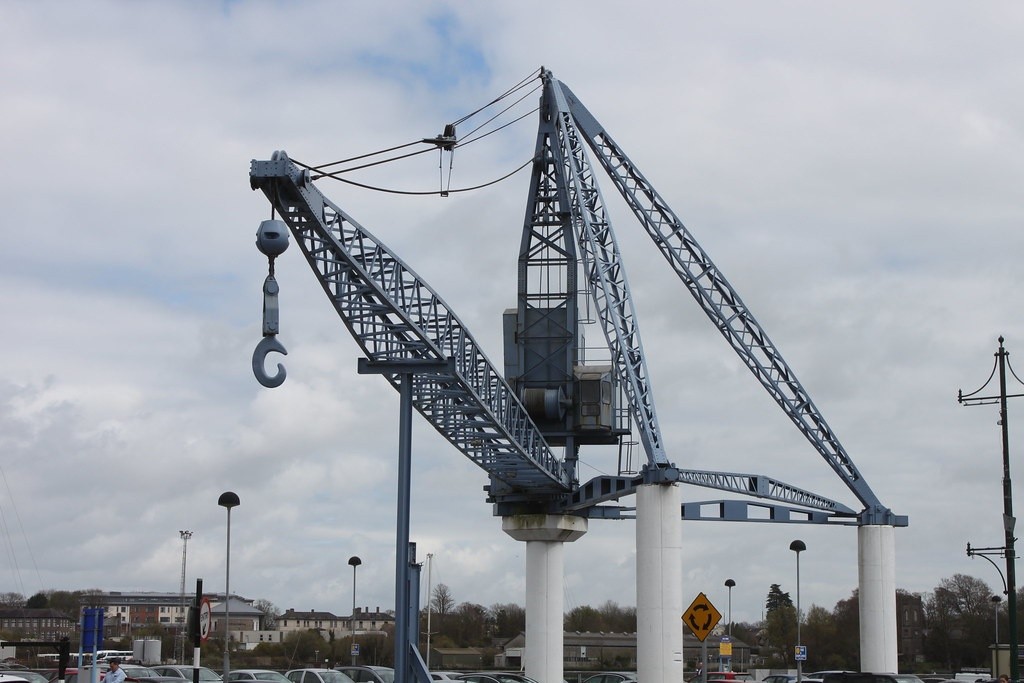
1;649;968;682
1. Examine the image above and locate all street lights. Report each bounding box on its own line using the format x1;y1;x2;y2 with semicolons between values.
725;577;737;671
789;538;807;683
347;555;364;664
217;490;240;680
991;595;1002;681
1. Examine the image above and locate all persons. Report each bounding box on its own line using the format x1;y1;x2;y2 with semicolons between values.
932;670;936;674
697;662;702;675
101;658;127;683
999;674;1009;683
